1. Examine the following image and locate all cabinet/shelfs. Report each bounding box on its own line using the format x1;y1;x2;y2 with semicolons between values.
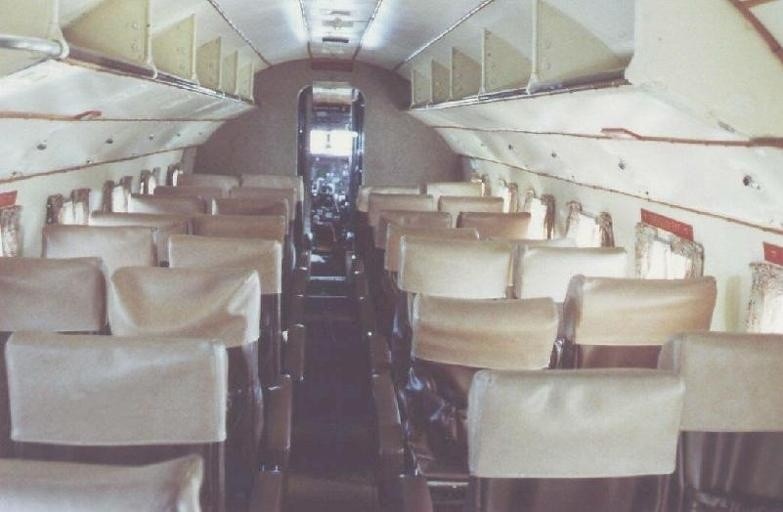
1;0;265;122
394;0;783;130
